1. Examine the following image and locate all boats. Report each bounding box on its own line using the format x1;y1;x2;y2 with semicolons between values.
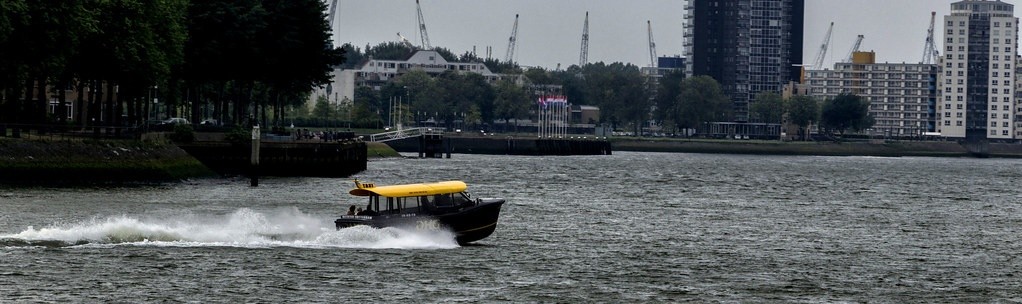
334;177;505;245
370;95;448;153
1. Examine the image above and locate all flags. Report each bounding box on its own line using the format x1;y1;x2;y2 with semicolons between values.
537;92;567;109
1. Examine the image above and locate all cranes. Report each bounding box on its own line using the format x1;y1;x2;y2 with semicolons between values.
327;0;342;47
579;11;589;66
646;20;657;67
504;13;519;63
922;11;936;65
415;0;432;50
843;34;865;63
810;21;834;70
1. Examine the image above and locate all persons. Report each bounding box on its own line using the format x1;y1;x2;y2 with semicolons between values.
346;204;380;216
433;193;456;208
296;128;337;142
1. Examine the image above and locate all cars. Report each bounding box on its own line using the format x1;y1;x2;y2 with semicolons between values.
612;131;674;138
157;118;190;125
201;119;225;126
